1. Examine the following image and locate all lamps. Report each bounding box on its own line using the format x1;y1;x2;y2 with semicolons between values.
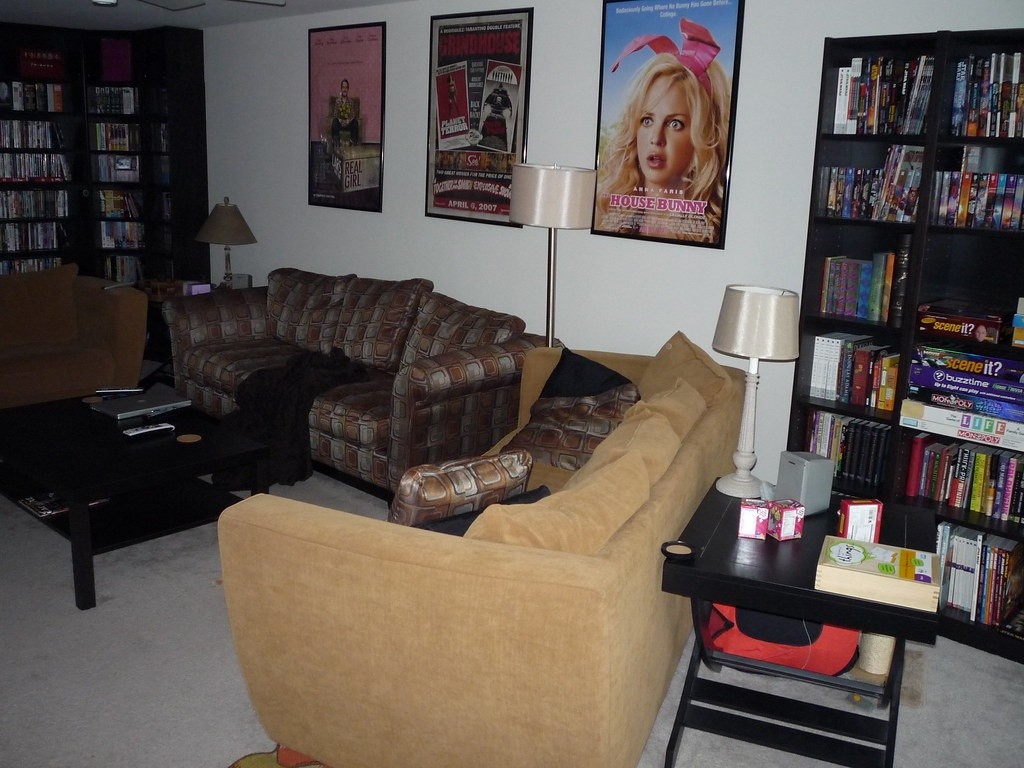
711;284;802;500
508;161;597;348
194;199;257;286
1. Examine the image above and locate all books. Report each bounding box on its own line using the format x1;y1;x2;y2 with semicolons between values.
814;535;941;613
100;220;149;249
797;330;900;412
927;166;1024;233
934;520;1024;641
96;153;140;183
899;340;1024;454
906;431;1024;524
0;118;66;149
812;143;924;222
830;51;933;136
0;257;63;277
820;231;913;329
950;50;1024;139
85;83;140;115
0;189;69;218
0;222;71;251
0;79;63;113
803;406;896;487
103;255;143;284
96;121;141;151
0;152;72;181
18;491;112;518
100;188;140;218
914;294;1024;350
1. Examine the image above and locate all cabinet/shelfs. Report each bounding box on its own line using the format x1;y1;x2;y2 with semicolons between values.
787;29;1024;666
0;21;208;290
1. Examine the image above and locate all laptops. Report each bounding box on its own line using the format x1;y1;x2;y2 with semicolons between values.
89;391;192;419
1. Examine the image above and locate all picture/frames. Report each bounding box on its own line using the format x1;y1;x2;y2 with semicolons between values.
307;20;387;213
425;7;534;228
591;0;746;250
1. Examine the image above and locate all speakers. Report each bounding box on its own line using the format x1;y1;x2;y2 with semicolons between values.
232;273;253;289
776;451;834;516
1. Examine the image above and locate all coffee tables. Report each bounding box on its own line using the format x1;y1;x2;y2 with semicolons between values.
0;394;267;610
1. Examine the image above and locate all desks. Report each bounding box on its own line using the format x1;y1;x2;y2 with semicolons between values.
660;477;936;767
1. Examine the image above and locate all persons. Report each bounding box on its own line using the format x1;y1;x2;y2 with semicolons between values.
594;14;732;242
446;73;463;116
331;78;361;153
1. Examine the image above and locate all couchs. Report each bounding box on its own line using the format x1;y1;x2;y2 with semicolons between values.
162;286;563;495
218;345;747;767
0;275;148;405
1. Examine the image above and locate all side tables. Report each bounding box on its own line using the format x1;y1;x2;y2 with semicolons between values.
144;295;174;360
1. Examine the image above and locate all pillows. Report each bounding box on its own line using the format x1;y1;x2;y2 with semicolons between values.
420;485;551;536
389;446;535;526
539;347;633;397
266;267;357;352
457;451;652;556
500;382;639;471
395;292;528;372
638;334;731;404
565;410;680;492
337;277;434;377
1;263;78;343
645;379;707;442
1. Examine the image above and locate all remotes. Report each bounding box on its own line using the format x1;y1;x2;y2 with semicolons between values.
101;281;135;290
122;423;175;438
95;388;144;396
